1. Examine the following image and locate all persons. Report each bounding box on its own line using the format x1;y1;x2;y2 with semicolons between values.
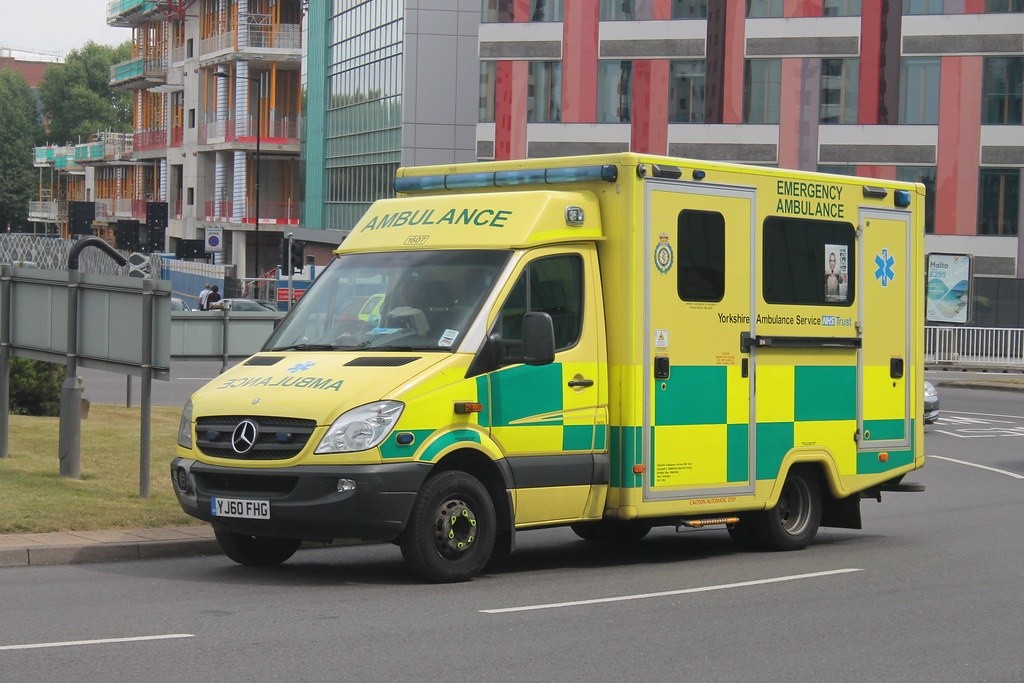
198;284;212;310
206;286;221;309
826;251;844;301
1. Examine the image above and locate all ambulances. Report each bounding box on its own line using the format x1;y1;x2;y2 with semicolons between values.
169;152;927;587
